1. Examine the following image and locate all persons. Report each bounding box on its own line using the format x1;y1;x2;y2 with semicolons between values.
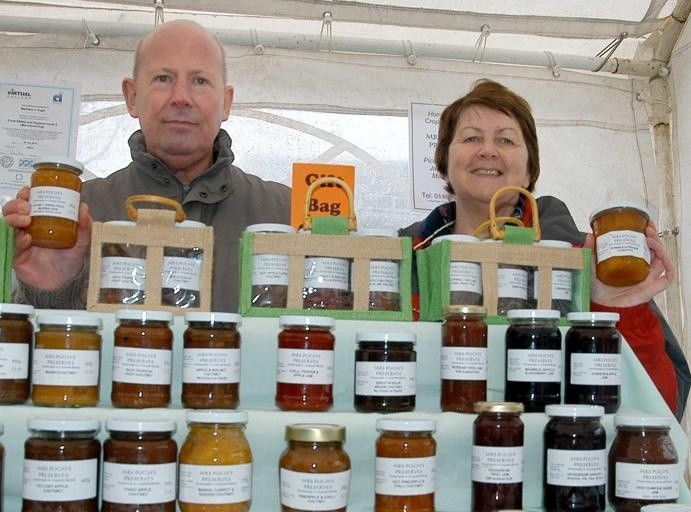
396;80;690;426
2;19;294;316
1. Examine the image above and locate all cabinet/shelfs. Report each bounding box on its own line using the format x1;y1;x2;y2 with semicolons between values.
0;309;690;512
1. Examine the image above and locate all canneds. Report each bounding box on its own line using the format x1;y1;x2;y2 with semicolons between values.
181;313;241;409
111;310;174;407
354;332;417;414
275;315;335;412
31;311;103;407
503;309;563;412
430;234;575;316
100;219;208;308
589;199;652;287
22;417;100;511
102;419;177;511
609;414;678;510
177;409;253;511
441;306;489;414
0;302;33;406
375;418;437;511
279;423;351;511
244;223;399;312
562;312;621;414
24;156;85;249
542;405;606;510
471;401;523;510
